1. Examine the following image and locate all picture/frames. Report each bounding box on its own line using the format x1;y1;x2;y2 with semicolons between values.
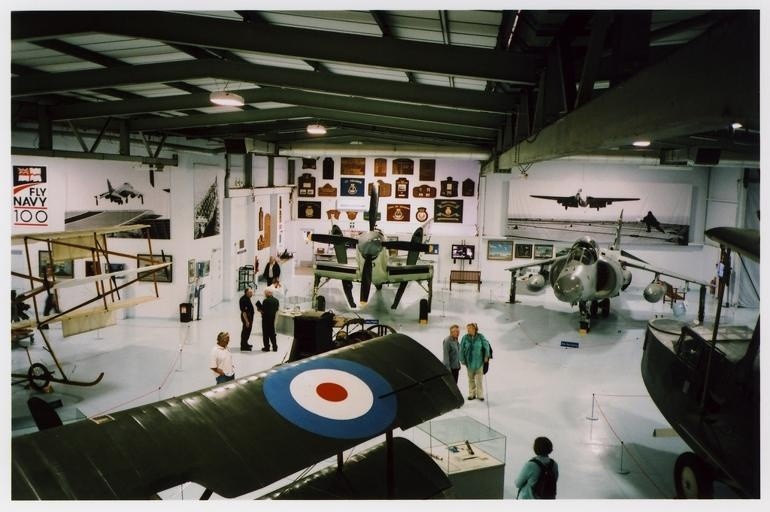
137;253;173;282
451;244;475;260
38;250;74;279
487;240;514;261
515;244;533;258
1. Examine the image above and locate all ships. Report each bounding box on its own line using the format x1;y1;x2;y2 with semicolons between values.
193;175;219;234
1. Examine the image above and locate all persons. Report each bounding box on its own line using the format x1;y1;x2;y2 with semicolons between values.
208;329;237;385
239;287;255;351
513;436;558;500
55;263;66;275
463;245;473;259
640;211;666;234
262;255;281;287
43;266;60;315
257;287;279;351
442;324;461;384
458;323;491;401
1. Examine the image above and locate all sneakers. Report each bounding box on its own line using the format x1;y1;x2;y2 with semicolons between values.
467;395;484;401
241;344;278;352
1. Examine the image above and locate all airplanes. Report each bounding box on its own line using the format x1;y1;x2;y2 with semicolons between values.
502;207;716;334
307;187;439;324
529;187;641;212
12;332;465;500
641;226;760;498
94;177;145;206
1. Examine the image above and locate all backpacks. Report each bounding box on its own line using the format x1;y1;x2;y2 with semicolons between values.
528;458;558;500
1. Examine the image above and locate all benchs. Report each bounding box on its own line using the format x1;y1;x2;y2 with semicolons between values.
449;269;481;292
661;279;686;308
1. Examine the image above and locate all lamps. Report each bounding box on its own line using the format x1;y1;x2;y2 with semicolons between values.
306;118;327;135
209;78;245;107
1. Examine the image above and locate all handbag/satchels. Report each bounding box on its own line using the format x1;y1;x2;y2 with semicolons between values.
479;332;494;375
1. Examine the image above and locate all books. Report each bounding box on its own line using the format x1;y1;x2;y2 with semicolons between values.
255;300;263;308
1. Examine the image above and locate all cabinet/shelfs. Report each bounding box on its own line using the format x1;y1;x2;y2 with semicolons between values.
414;415;507;499
238;265;258;291
